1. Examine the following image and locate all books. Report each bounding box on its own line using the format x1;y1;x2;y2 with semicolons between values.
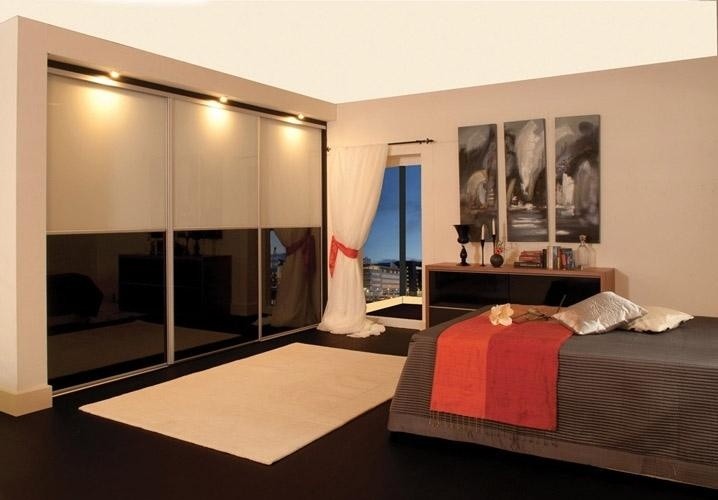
514;246;575;271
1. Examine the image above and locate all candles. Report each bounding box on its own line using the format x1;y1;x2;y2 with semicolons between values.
491;217;496;235
481;224;485;239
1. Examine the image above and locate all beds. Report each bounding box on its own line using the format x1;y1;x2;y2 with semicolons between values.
387;303;717;491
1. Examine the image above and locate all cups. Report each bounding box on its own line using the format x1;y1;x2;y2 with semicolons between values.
452;224;473;245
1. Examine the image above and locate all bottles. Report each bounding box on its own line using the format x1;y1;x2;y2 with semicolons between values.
574;234;594;266
541;249;547;270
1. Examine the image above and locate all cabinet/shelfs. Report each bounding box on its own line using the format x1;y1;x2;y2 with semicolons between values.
424;262;615;328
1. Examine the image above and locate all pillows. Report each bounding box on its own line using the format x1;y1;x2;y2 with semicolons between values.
551;291;648;335
621;305;694;333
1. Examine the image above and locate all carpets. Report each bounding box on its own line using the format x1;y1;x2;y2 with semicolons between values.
78;341;407;466
